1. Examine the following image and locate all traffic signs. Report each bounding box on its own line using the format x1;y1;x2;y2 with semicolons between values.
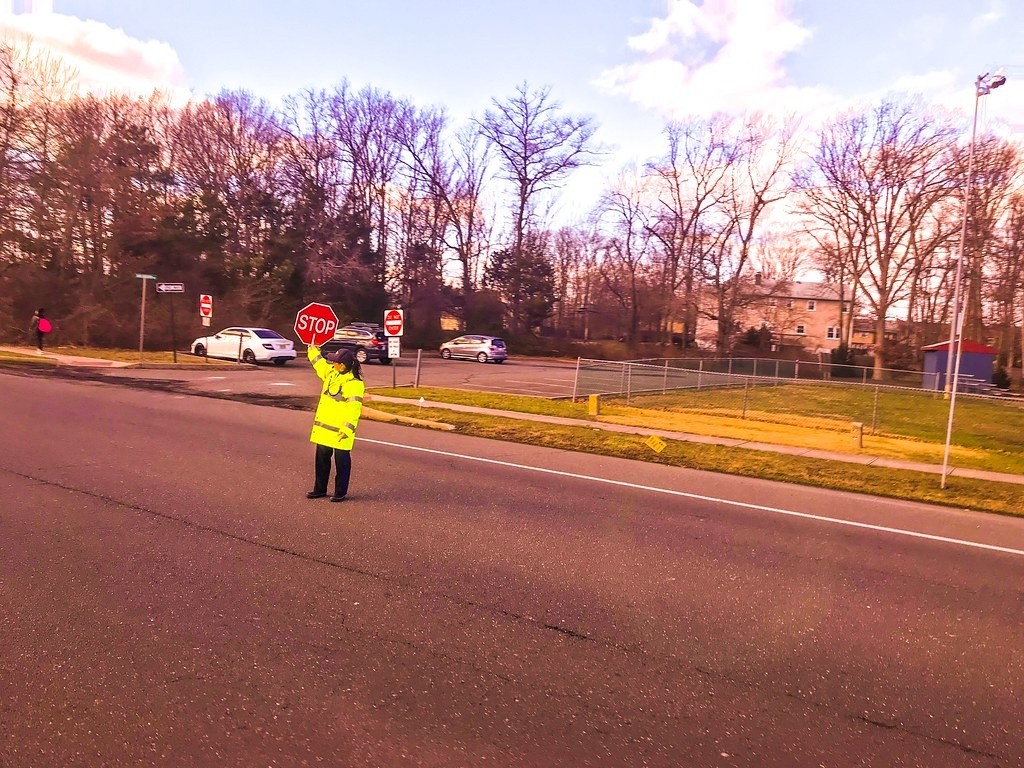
155;282;185;295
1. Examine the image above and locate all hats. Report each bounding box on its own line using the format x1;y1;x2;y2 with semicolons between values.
328;349;353;362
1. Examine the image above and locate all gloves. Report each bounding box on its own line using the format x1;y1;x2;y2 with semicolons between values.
307;344;315;352
337;431;348;442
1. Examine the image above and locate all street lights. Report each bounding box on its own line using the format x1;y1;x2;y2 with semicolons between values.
940;74;1008;489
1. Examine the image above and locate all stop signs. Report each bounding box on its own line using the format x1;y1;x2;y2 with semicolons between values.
293;303;340;348
199;296;212;318
384;310;403;336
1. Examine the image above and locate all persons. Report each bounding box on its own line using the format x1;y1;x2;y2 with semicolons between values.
29;304;45;355
305;343;364;502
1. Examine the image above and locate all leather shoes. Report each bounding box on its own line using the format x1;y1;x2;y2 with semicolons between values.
330;495;345;502
307;492;326;498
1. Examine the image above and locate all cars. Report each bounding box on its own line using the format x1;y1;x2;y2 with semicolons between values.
439;334;509;364
191;327;298;364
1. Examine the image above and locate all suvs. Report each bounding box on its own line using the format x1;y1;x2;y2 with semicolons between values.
307;322;403;364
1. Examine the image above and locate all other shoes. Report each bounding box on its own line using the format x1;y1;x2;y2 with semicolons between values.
36;349;44;355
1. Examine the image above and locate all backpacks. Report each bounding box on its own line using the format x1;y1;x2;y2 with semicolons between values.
34;316;52;333
